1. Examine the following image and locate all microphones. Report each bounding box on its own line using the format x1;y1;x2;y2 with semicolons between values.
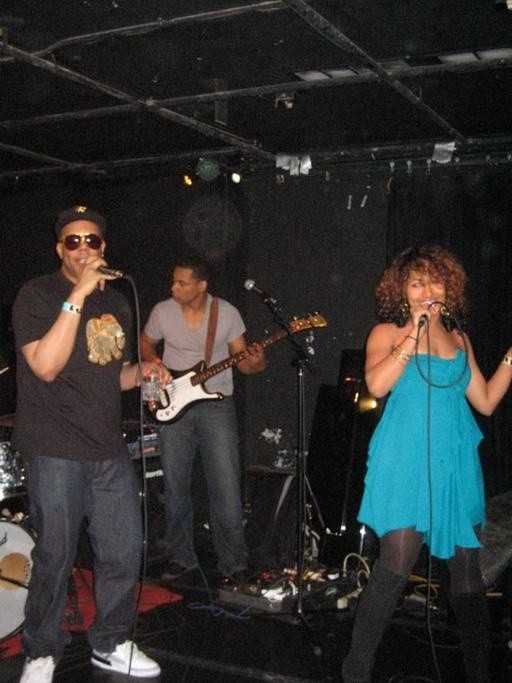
242;277;278;309
96;263;125;278
417;300;436;329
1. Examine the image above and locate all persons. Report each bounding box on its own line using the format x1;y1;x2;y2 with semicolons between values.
140;259;266;591
339;245;512;683
10;205;172;683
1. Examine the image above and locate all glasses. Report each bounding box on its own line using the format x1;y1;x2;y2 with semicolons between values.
62;233;103;250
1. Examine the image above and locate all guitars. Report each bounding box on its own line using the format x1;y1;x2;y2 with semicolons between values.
147;309;327;425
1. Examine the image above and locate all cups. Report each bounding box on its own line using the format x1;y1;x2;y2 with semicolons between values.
0;441;23;489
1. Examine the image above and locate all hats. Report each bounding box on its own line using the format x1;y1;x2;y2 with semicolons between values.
53;205;109;239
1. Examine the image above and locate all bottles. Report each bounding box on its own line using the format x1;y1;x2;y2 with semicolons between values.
418;602;447;615
143;375;160;401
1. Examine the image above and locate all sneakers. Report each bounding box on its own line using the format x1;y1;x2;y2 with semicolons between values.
150;557;261;590
19;655;57;683
90;639;162;679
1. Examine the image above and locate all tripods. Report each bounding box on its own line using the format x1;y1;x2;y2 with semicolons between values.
244;405;353;657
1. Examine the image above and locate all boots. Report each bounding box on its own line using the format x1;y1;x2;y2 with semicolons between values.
448;589;504;683
342;559;409;683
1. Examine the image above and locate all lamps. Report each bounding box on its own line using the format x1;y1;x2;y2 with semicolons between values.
428;141;457;163
269;149;316;177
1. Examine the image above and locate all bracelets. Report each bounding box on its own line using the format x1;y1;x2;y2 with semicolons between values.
62;301;83;316
501;356;512;367
390;345;412;366
